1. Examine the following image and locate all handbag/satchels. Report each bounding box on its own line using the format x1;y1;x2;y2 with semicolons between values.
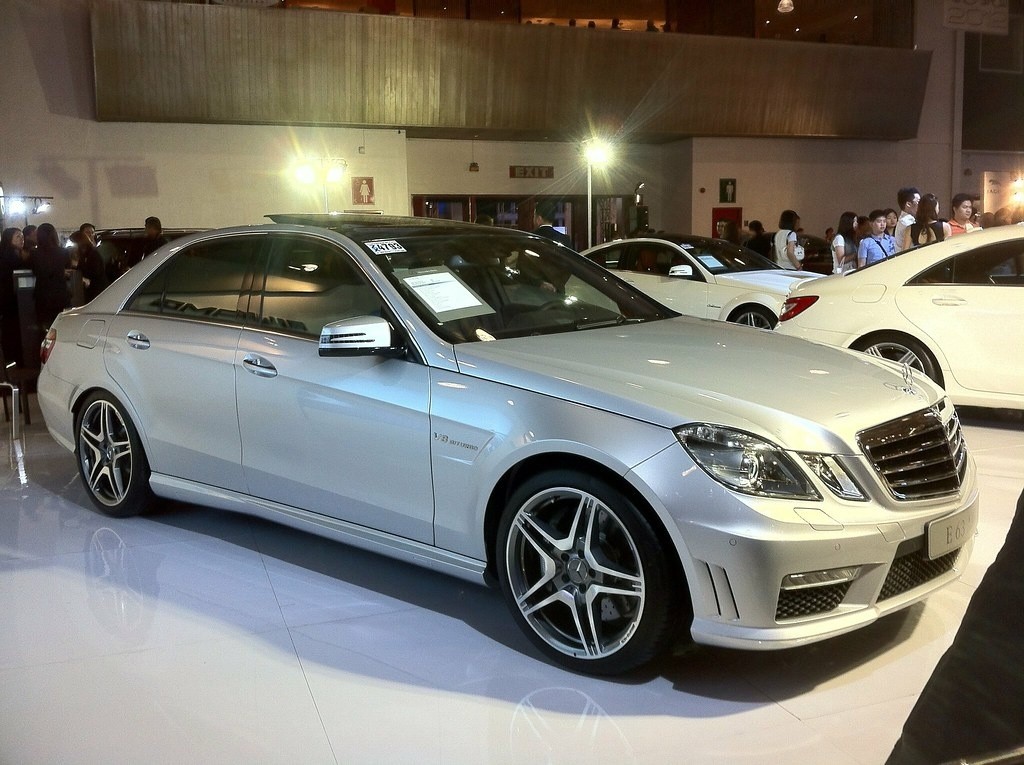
779;231;805;262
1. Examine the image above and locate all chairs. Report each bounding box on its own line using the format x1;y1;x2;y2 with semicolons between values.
0;344;40;425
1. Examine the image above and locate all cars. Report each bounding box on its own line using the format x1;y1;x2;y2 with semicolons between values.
37;212;978;678
771;225;1022;412
556;230;832;329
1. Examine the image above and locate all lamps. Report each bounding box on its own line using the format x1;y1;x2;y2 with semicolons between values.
777;0;794;13
32;197;51;214
470;163;479;171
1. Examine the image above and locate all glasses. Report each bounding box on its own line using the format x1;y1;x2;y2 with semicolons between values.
929;193;936;202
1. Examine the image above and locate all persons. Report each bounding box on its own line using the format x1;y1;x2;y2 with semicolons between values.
468;214;494;267
0;223;72;368
133;217;168;268
825;186;1023;276
608;225;666;242
69;224;110;308
516;197;574;296
717;210;811;271
